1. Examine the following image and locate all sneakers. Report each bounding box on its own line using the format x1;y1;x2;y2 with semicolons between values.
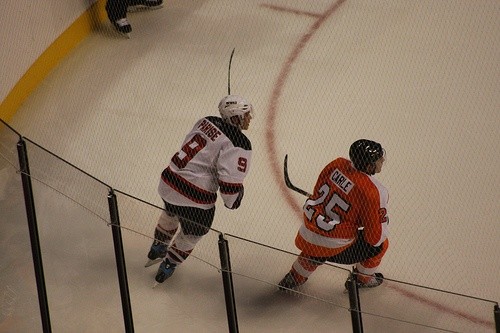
154;259;176;288
342;272;386;294
107;0;164;40
144;241;169;268
276;273;309;293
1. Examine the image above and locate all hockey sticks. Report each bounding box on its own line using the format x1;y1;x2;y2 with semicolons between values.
283;154;312;197
228;48;236;95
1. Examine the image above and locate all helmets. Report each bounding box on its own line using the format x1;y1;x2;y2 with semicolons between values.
349;139;386;172
218;95;252;127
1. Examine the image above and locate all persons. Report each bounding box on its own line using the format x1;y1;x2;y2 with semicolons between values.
277;139;390;292
144;94;252;289
105;0;164;38
